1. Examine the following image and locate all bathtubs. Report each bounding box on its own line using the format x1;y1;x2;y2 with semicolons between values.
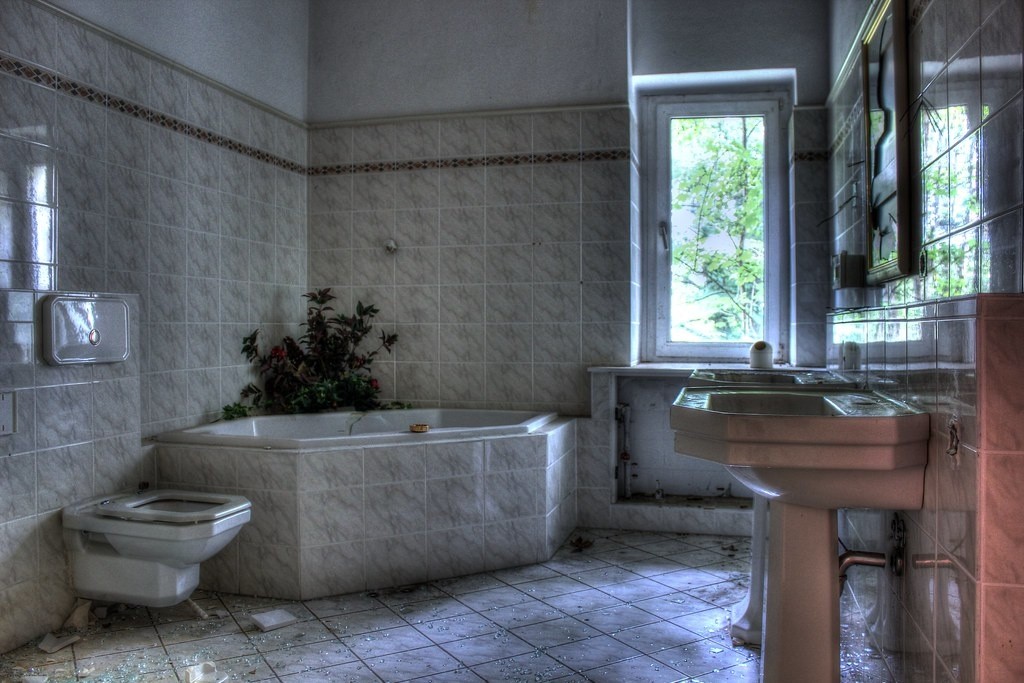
155;406;576;602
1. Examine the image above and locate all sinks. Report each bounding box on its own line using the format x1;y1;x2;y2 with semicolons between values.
685;365;810;388
669;385;930;518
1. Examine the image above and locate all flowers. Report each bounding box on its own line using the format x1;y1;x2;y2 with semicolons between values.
210;287;413;424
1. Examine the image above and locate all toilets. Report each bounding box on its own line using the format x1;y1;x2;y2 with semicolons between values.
62;489;252;606
